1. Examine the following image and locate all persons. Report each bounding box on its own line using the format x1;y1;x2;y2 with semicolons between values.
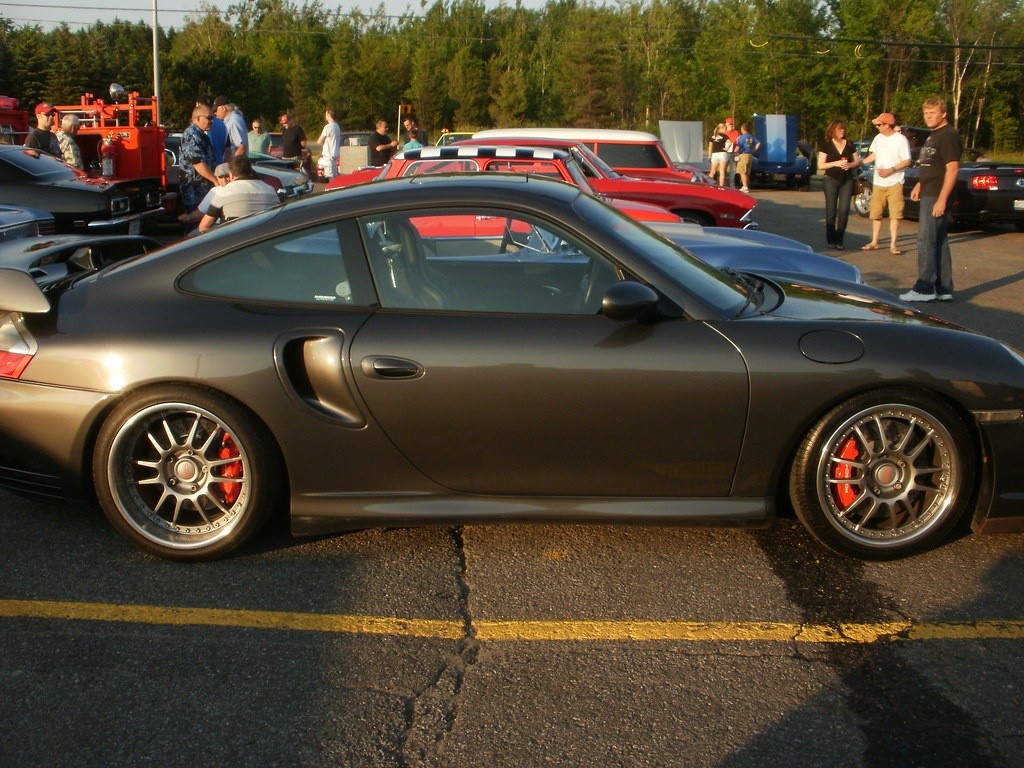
317;108;340;182
25;101;62;159
797;141;817;175
178;162;230;239
198;156;281;232
280;114;307;166
369;119;398;166
818;120;859;249
708;118;761;193
900;99;963;302
247;119;272;155
403;130;425;151
179;95;249;236
55;113;83;170
403;117;428;146
857;113;913;255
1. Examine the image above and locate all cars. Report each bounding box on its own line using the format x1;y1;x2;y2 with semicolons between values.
435;132;475;146
0;171;1024;561
0;144;166;234
0;205;165;285
752;114;811;189
325;137;759;231
372;146;683;237
853;147;1024;232
164;132;314;203
316;132;397;181
470;128;719;186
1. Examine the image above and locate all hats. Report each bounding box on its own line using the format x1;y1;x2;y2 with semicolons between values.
725;118;733;123
34;101;60;116
212;95;228;111
871;113;895;125
195;95;214;109
214;162;230;176
279;114;292;123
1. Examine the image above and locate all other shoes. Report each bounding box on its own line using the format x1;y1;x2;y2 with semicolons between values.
739;186;750;193
827;242;844;250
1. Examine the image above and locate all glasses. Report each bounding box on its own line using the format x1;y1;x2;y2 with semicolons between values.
40;113;56;116
218;174;230;178
877;124;886;126
254;127;261;129
198;114;214;119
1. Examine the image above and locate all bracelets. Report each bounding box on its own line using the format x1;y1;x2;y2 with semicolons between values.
890;167;896;172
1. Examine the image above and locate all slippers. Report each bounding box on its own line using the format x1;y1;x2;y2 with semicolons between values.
859;244;880;251
889;248;902;255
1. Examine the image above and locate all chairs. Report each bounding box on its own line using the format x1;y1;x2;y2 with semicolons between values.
372;219;458;309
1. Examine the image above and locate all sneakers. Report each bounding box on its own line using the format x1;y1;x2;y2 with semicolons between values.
899;290;936;302
936;292;953;302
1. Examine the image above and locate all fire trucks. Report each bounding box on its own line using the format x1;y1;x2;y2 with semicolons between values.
0;83;178;225
